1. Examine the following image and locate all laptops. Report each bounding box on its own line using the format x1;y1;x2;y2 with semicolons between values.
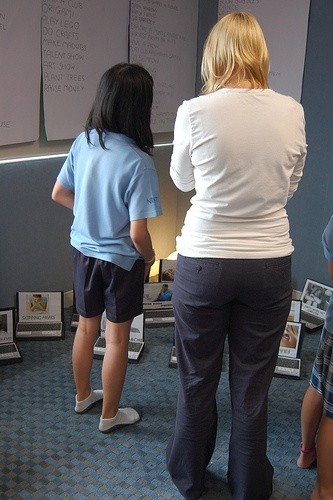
0;259;333;380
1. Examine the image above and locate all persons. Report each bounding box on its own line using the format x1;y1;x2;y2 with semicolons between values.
166;12;308;500
153;283;172;302
304;286;324;309
52;64;161;433
28;294;48;312
296;215;333;500
